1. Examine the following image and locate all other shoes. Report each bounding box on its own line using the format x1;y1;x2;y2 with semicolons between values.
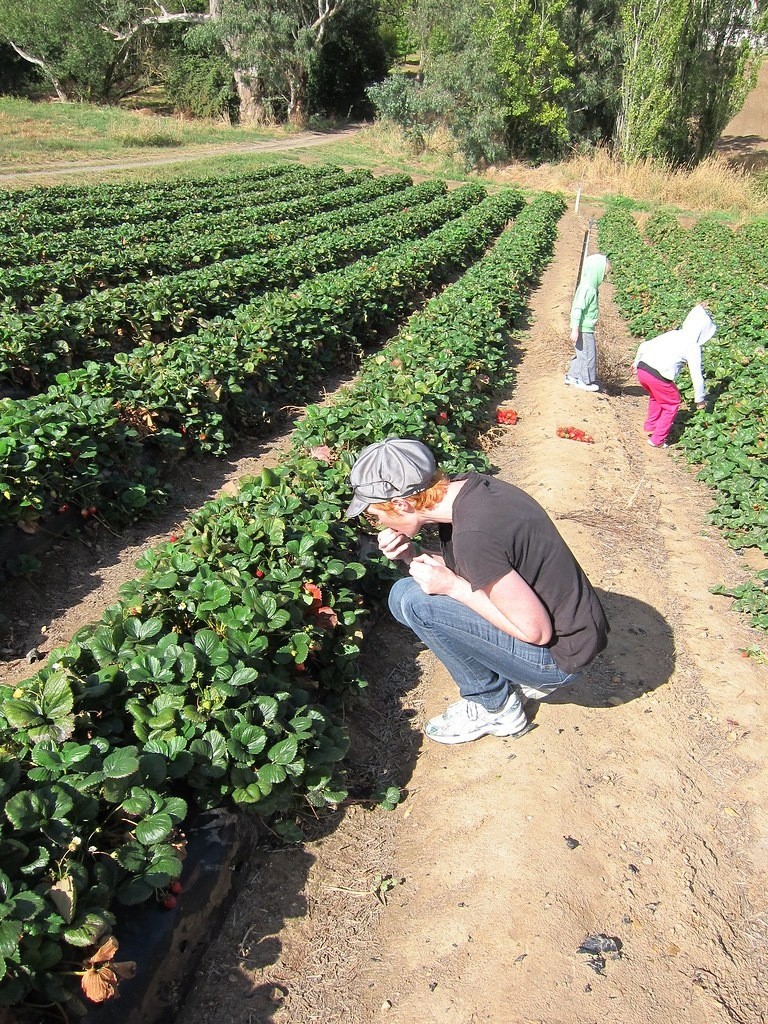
564;374;578;385
578;380;600;391
646;429;655;437
647;440;666;448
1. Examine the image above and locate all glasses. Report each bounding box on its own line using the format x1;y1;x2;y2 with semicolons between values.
344;476;399;494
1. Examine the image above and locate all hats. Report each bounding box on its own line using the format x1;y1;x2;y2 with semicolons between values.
345;437;435;518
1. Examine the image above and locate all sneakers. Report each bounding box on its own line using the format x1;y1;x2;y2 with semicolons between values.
507;677;558;708
425;691;527;744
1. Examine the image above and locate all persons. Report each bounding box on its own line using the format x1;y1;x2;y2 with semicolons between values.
344;439;612;745
630;304;717;448
565;254;612;391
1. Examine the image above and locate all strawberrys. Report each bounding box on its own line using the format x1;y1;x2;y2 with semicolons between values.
182;427;187;433
294;639;322;672
742;651;748;657
496;409;517;425
439;412;447;419
49;489;96;518
255;570;264;577
556;426;593;443
169;536;177;542
162;881;182;909
